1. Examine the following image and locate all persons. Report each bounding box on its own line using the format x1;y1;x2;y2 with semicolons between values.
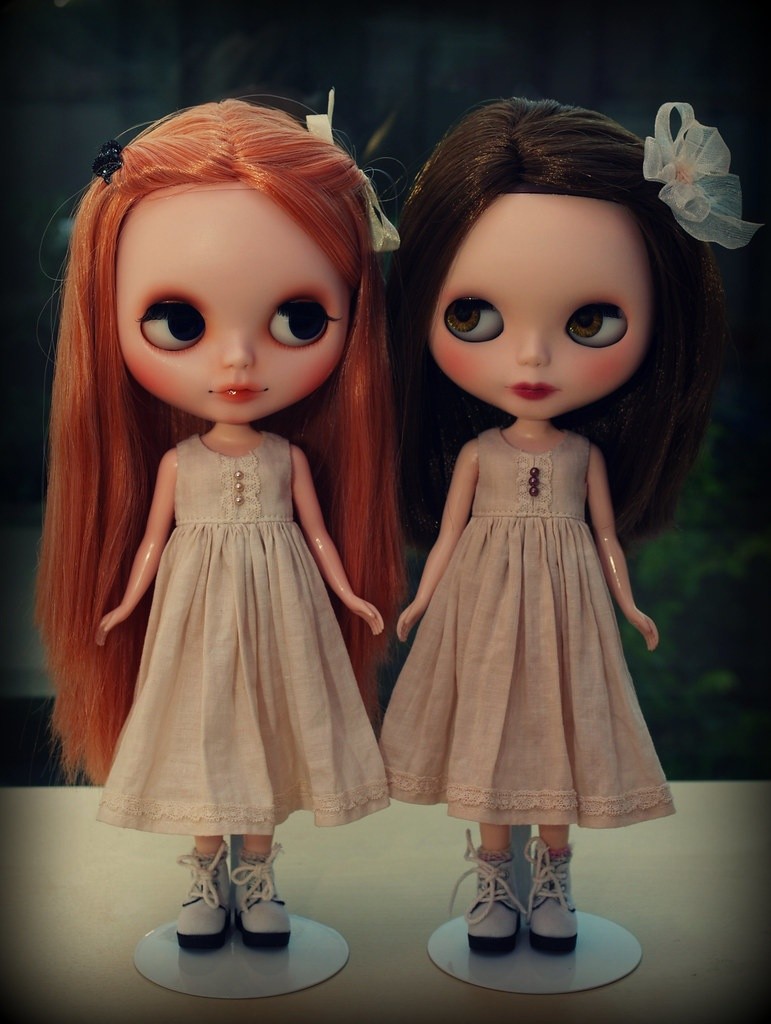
384;96;730;957
30;98;408;950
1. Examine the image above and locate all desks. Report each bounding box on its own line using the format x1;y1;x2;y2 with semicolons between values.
0;781;771;1024
